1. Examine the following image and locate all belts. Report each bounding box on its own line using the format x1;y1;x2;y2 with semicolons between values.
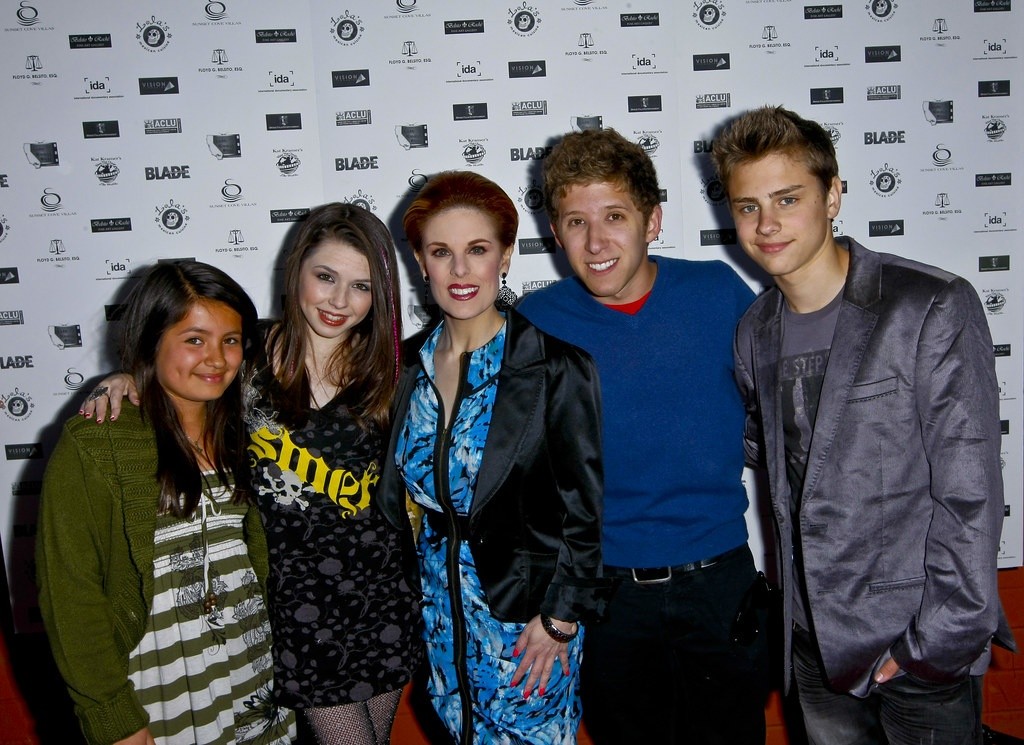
602;542;749;584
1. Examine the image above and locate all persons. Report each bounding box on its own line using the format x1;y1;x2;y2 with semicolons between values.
373;169;607;745
34;261;306;745
76;200;424;745
512;126;782;745
707;104;1017;745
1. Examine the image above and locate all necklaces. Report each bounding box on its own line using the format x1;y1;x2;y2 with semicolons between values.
179;419;206;452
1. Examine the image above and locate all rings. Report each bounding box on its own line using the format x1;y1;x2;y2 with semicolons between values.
89;386;111;403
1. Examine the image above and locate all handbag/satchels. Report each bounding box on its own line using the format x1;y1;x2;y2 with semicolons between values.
730;571;778;662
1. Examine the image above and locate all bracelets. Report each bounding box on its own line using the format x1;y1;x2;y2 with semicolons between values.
540;613;579;643
